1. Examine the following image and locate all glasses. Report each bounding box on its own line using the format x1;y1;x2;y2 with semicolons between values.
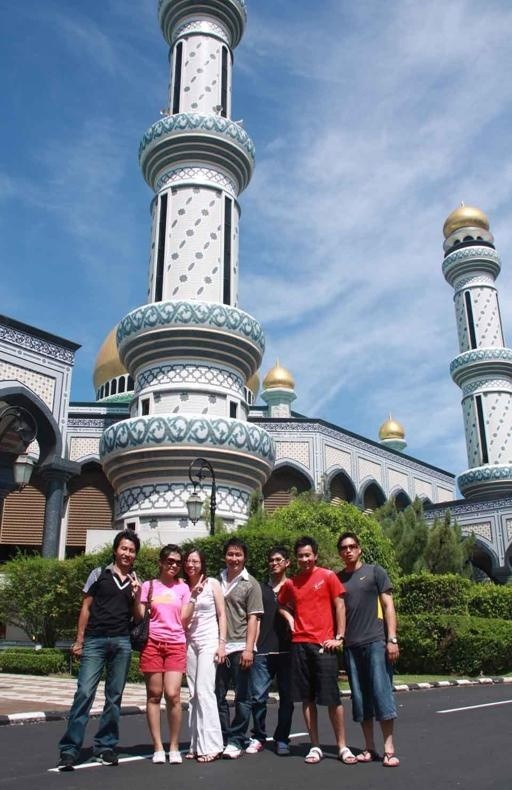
341;544;357;550
166;558;183;566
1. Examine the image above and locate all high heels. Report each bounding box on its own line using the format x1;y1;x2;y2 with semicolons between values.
197;752;222;763
185;753;197;759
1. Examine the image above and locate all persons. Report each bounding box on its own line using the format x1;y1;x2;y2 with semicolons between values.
332;532;400;765
244;547;294;755
126;543;210;764
56;531;143;771
215;536;264;759
182;544;228;764
277;536;358;764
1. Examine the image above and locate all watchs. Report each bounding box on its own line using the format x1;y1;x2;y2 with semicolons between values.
335;634;344;640
388;637;398;644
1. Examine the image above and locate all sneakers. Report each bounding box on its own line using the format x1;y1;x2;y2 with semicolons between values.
56;753;82;771
94;750;118;766
275;741;290;755
245;737;267;754
222;744;244;759
169;751;183;764
152;750;166;764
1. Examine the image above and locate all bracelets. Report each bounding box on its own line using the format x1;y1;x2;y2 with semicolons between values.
189;597;197;602
219;638;227;643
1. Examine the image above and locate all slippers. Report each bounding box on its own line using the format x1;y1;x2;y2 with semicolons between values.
383;753;400;767
356;750;378;762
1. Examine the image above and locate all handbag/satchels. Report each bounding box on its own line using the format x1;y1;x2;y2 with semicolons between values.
131;612;151;650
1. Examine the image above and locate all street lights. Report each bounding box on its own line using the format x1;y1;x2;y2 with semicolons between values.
186;458;217;537
0;399;38;533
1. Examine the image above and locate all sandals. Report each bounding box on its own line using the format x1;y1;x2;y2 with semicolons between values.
304;747;324;763
338;747;358;764
